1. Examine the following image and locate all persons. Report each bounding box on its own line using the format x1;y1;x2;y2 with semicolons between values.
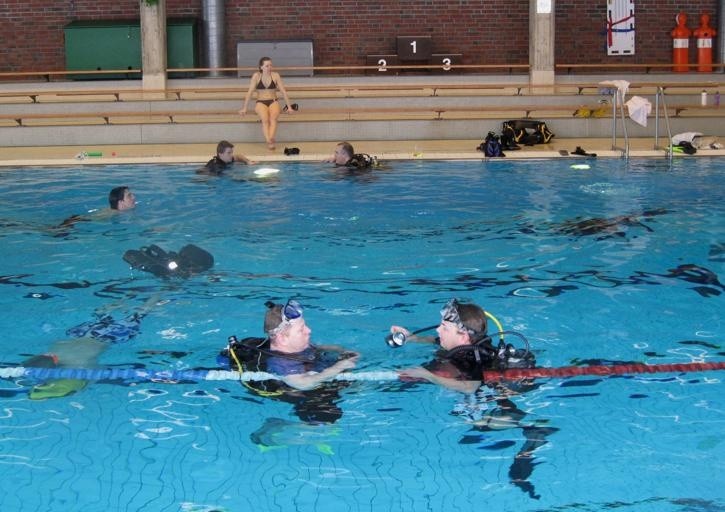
250;302;362;455
297;141;391;186
0;184;178;242
14;284;170;394
237;56;299;150
197;141;258;181
389;298;547;442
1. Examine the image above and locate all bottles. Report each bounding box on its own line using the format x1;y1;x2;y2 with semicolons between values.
713;92;721;106
701;89;708;106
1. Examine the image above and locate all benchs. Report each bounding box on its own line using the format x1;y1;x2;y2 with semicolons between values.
0;83;725;145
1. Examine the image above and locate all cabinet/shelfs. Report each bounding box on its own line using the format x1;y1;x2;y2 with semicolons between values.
62;18;199;77
236;38;314;78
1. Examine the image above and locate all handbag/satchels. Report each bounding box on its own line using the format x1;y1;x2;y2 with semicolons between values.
484;131;505;157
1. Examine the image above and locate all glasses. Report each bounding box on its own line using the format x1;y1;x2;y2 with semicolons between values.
264;299;304;335
439;298;487;336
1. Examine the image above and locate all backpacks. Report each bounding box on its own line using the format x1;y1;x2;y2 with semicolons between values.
503;120;554;144
